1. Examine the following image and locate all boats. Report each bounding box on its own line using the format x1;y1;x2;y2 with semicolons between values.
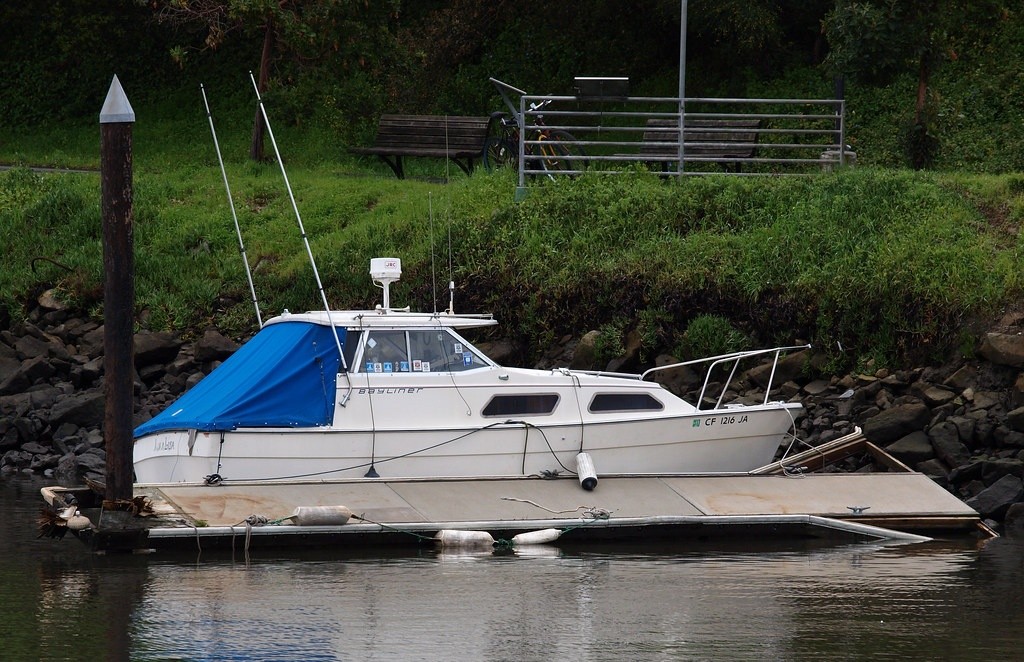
39;71;981;552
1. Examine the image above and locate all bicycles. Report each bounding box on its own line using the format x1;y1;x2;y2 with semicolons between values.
484;93;590;187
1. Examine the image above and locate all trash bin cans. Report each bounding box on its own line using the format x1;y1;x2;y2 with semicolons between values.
822;151;856;174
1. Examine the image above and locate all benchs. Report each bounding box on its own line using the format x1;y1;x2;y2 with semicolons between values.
348;114;490;183
614;120;759;182
